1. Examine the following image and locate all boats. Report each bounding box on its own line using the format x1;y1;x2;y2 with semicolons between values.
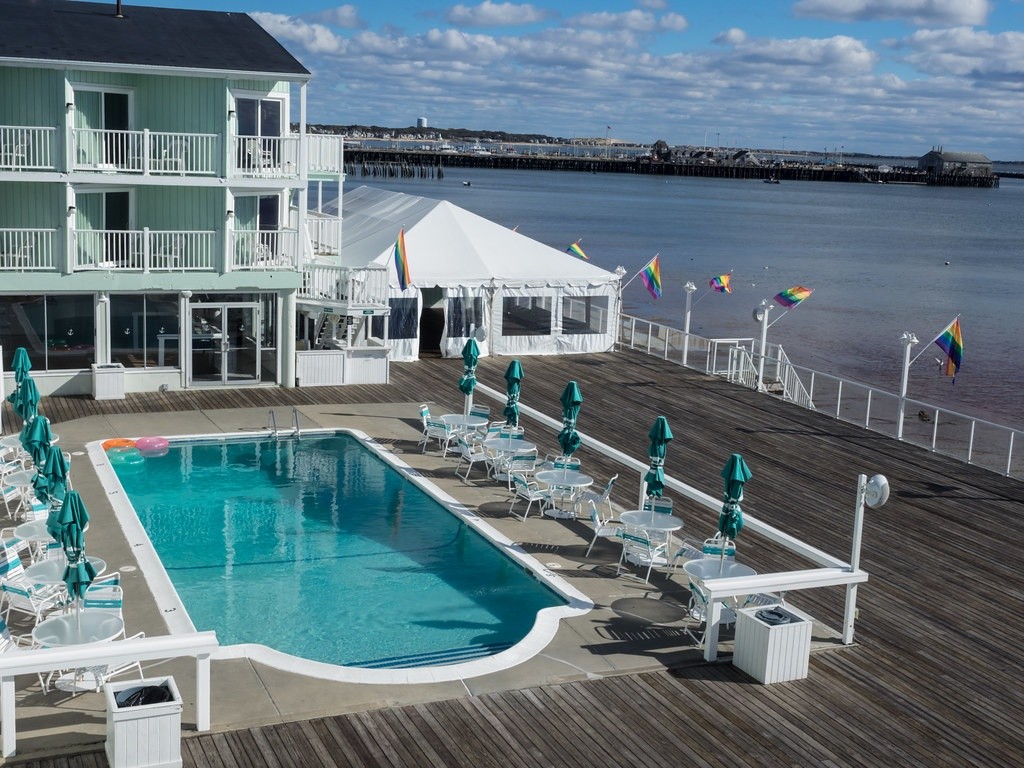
764;176;781;184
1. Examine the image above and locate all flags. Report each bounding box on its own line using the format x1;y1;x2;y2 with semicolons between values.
565;241;592;261
773;287;813;310
709;273;732;294
395;228;411;290
935;319;962;386
640;256;662;300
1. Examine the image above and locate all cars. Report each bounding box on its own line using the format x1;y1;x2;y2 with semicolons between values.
699;159;717;165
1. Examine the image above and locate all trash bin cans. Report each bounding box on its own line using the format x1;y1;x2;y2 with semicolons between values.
732;604;812;684
91;363;126;400
104;675;183;768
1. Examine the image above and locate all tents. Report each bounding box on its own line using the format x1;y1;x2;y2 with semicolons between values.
313;186;622;361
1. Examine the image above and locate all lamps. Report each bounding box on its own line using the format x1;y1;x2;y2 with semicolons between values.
67;103;74;111
69;206;76;214
227;210;233;217
228;110;236;117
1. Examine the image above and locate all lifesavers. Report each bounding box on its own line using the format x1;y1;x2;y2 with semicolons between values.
102;439;135;451
140;447;168;457
136;438;169;450
114;466;147;476
109;455;146;467
105;446;141;458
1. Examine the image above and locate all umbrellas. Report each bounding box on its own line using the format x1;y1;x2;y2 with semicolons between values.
458;338;481;419
22;416;52;515
557;381;583;478
57;490;96;629
718;453;752;569
12;348;32;414
44;445;69;566
503;360;524;444
17;376;40;468
644;416;673;520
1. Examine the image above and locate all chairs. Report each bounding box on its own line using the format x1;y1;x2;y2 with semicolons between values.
244;234;272;267
129;137;189;176
0;133;31;168
134;233;187;269
246;140;272;171
0;416;145;705
416;401;790;647
4;234;36;268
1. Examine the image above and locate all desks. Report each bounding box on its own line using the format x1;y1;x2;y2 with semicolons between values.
31;613;124;693
13;517;89;557
1;429;59;465
619;512;685;567
4;469;38;520
534;470;594;519
681;558;758;625
24;555;106;620
439;412;489;452
482;438;537;480
